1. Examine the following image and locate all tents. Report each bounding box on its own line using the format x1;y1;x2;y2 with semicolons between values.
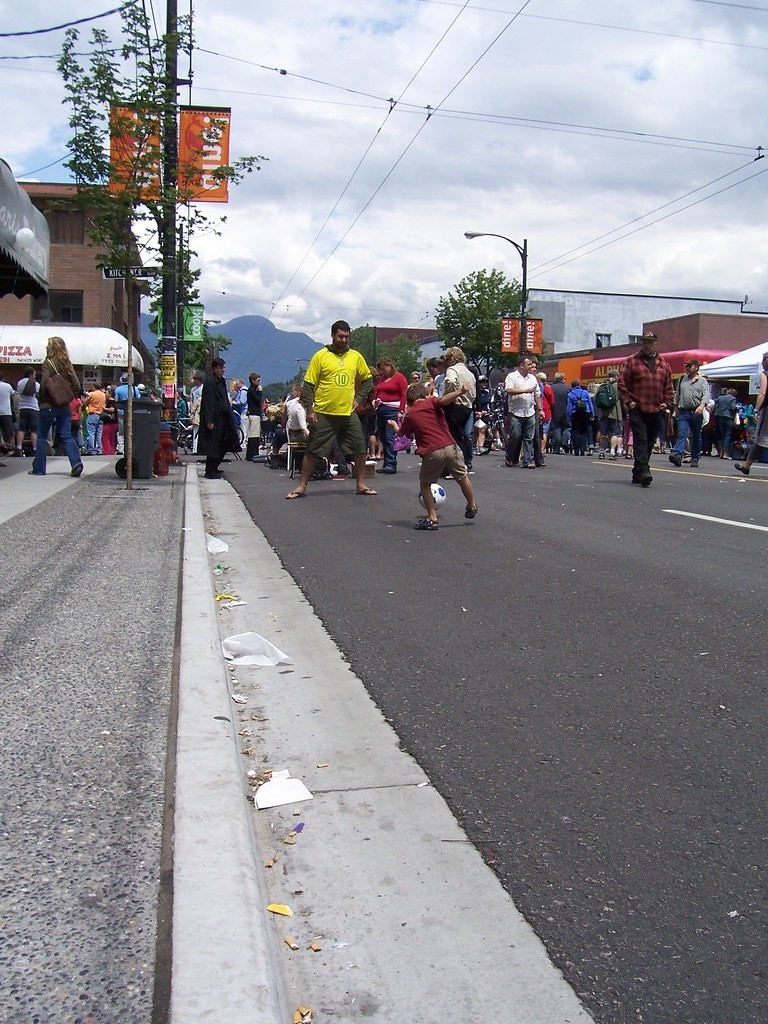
696;342;768;385
579;348;754;392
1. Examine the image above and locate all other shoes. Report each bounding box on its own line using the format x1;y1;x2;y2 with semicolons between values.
28;470;33;474
415;518;440;530
204;469;225;479
669;454;699;467
71;462;83;477
735;462;750;475
599;450;634;459
465;503;479;519
337;464;352;476
376;466;397;474
473;446;500;454
631;472;653;486
367;455;384;461
520;459;546;468
6;448;23;457
443;467;476;479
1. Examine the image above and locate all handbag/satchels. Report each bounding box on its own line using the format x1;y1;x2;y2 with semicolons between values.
99;411;115;422
44;358;75;407
393;416;410;452
734;412;742;425
658;406;675;442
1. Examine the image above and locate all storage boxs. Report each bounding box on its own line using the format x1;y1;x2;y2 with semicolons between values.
349;460;377;480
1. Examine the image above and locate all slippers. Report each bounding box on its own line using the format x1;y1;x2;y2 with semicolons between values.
357;488;379;495
286;490;306;498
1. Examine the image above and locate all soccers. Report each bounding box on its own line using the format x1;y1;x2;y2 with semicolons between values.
418;483;447;511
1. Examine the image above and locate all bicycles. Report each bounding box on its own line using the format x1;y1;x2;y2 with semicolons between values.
167;415;194;449
482;401;507;454
235;426;244;446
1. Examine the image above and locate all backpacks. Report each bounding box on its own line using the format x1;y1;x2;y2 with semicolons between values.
573;389;587;412
595;379;620;410
21;379;38;397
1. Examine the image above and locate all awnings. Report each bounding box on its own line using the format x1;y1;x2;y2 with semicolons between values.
1;156;54;299
0;322;145;376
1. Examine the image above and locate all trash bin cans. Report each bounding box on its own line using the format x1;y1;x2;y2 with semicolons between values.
114;400;162;479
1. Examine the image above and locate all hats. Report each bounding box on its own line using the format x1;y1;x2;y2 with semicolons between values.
684;359;700;365
478;375;491;381
119;373;129;384
639;331;658;342
609;370;619;377
536;372;547;380
137;384;145;390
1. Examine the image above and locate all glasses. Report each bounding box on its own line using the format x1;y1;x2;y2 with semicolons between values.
414;377;420;379
257;378;260;381
685;364;692;368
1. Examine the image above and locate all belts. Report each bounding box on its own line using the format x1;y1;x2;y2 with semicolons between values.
87;412;101;417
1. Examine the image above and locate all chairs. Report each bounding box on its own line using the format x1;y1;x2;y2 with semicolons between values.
284;419;329;479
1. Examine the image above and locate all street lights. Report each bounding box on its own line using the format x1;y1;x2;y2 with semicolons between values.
464;230;528;360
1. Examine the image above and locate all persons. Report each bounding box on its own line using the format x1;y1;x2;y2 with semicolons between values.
285;319;382;499
0;336;768;482
618;331;676;485
385;376;481;531
